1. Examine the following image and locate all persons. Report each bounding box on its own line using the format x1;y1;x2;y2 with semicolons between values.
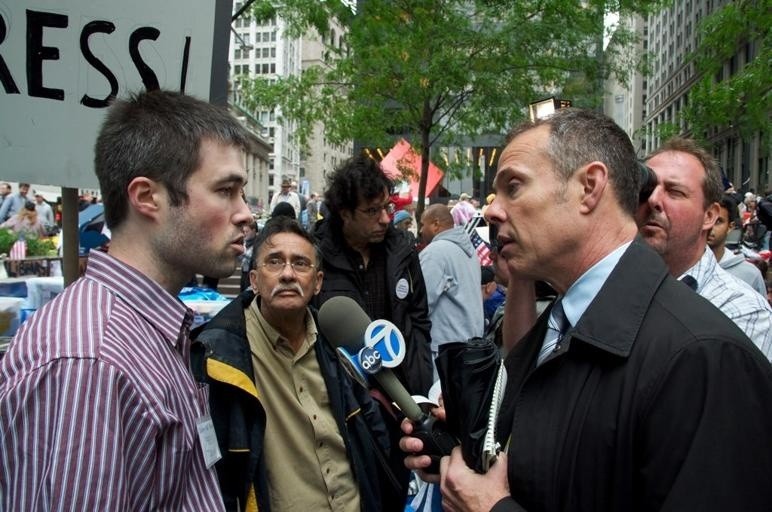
0;87;255;512
635;135;772;360
189;217;418;512
391;110;772;512
0;155;771;512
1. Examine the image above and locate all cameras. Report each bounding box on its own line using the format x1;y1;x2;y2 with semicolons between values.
638;161;657;204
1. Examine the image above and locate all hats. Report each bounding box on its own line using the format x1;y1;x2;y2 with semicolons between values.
481;265;496;285
280;179;291;187
56;230;111;258
394;209;411;224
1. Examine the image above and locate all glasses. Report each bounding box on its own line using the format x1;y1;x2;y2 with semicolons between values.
254;257;315;274
363;202;396;219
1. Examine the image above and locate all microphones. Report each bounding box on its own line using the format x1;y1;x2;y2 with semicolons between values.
318;296;460;461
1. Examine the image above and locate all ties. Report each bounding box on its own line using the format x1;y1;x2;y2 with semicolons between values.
536;298;568;368
